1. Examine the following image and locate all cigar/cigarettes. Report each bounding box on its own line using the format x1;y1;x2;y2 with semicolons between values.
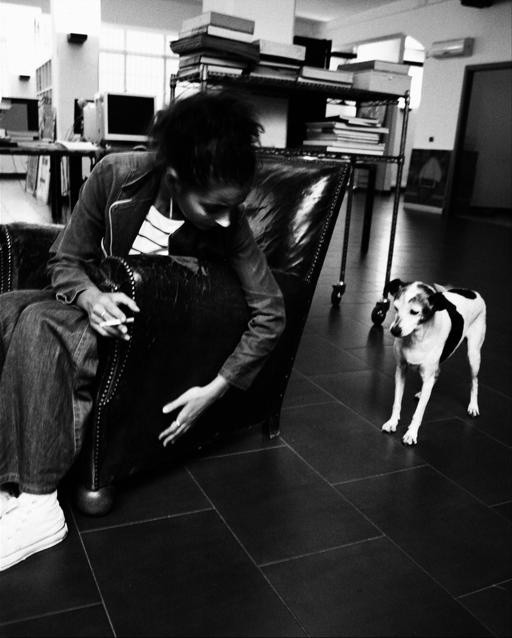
100;315;135;328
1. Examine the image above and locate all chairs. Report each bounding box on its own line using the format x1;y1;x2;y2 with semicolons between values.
0;147;353;515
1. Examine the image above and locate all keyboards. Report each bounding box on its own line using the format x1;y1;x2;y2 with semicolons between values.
10;141;67;148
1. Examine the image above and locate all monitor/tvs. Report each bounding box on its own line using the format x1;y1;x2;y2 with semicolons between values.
0;97;41;136
100;90;157;142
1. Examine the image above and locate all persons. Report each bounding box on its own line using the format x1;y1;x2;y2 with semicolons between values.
1;91;286;577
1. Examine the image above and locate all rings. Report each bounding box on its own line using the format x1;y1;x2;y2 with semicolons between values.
175;418;182;428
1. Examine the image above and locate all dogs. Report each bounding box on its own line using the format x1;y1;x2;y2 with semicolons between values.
382;277;489;446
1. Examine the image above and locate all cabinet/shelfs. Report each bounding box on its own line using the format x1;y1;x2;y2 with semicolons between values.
33;31;98;197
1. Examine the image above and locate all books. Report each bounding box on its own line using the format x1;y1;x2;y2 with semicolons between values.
169;10;414;160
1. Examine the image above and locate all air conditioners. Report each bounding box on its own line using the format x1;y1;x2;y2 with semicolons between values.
426;37;474;59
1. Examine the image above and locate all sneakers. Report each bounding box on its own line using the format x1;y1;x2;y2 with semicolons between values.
2;490;67;569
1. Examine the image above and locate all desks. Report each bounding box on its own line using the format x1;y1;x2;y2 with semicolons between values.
169;68;410;286
1;139;101;224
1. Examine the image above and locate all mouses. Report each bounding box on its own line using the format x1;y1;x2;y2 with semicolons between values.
131;144;147;151
